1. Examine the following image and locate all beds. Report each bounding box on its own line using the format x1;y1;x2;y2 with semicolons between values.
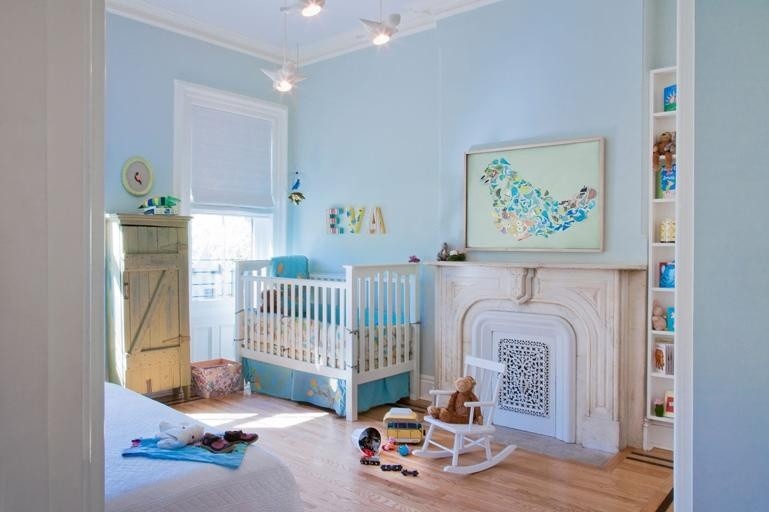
233;259;420;423
104;379;303;512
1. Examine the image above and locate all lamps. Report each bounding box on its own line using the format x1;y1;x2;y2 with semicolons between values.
300;0;326;17
260;7;308;93
360;0;401;46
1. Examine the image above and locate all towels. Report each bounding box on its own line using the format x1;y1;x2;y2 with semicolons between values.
271;256;309;299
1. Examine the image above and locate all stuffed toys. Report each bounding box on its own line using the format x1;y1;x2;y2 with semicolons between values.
428;376;484;424
653;131;674;172
652;299;668;330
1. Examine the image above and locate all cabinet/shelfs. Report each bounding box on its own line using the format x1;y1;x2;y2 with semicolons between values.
643;67;679;423
106;213;193;401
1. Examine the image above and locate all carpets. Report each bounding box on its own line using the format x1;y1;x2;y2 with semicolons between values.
421;419;612;468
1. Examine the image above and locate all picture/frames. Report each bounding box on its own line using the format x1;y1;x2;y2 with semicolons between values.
462;137;605;254
122;157;154;195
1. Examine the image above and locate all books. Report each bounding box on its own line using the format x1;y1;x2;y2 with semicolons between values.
656;342;674;375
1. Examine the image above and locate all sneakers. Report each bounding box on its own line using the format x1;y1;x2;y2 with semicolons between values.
224;430;258;443
202;432;234;453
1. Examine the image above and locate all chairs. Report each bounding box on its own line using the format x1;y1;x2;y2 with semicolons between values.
411;356;516;474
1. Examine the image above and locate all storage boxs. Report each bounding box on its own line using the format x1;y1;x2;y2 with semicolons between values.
191;358;244;399
